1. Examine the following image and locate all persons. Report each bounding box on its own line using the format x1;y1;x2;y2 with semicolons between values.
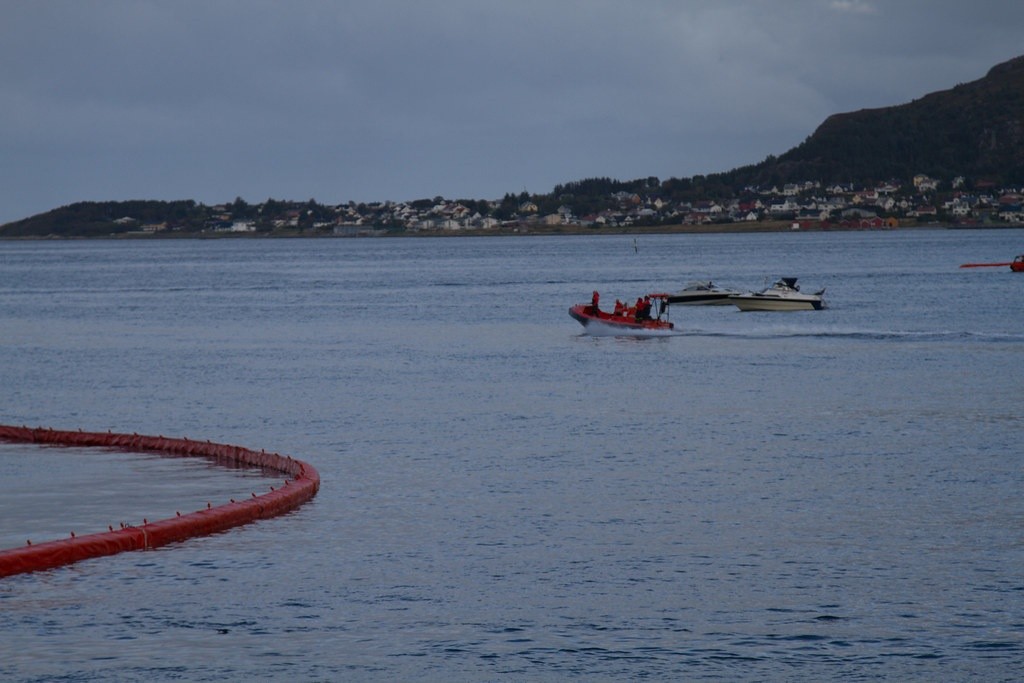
636;297;651;324
590;290;599;317
614;300;623;316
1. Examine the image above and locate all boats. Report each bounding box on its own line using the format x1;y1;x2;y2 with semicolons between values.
667;282;742;305
728;277;828;312
569;291;675;331
1010;255;1024;272
